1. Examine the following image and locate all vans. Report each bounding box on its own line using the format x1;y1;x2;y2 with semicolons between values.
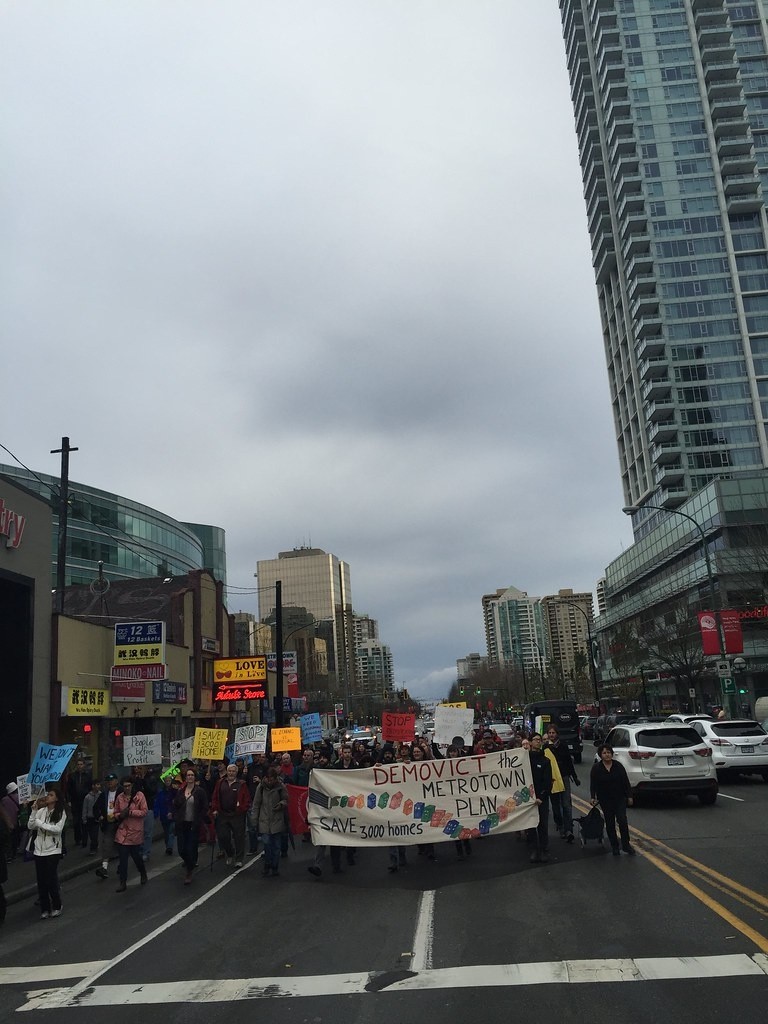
518;699;583;764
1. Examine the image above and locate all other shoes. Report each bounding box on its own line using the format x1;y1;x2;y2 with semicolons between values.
567;831;574;842
273;869;280;876
52;905;64;918
142;853;150;861
40;910;50;918
612;849;621;856
556;824;565;839
399;859;406;866
247;847;258;855
333;868;343;874
623;844;635;854
308;864;322;876
418;851;424;856
182;861;199;872
543;851;550;861
428;853;435;859
387;863;397;871
529;848;539;863
166;848;173;855
281;852;288;857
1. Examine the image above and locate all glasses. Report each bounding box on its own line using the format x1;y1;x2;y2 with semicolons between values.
533;738;543;741
77;764;82;765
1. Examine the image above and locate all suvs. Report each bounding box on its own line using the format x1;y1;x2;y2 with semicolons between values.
688;718;768;782
593;720;719;806
350;728;375;751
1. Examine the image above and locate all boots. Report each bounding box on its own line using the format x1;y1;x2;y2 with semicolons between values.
117;880;126;891
456;842;472;860
302;832;311;842
141;873;148;884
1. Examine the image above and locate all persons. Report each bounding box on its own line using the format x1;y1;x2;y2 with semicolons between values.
24;790;67;919
286;698;302;711
590;744;638;855
0;724;581;892
717;707;724;720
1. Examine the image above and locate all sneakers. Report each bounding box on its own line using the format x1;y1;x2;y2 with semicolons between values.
226;857;232;866
89;846;97;855
96;866;109;878
235;862;242;867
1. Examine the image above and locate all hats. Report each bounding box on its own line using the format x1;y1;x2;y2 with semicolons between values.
103;773;118;780
6;781;18;794
379;743;396;756
483;732;493;738
92;779;99;784
178;757;194;769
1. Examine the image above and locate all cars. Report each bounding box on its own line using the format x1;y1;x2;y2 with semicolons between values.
423;721;435;733
322;729;339;743
511;717;523;732
486;724;515;744
346;730;354;742
579;715;667;738
482;717;494;726
664;712;713;723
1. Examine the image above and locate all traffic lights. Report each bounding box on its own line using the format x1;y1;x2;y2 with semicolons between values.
460;686;464;696
477;686;481;694
735;673;749;694
508;703;511;711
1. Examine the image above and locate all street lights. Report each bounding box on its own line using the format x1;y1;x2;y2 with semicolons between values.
549;601;601;716
512;636;546;701
622;505;740;721
500;650;528;703
